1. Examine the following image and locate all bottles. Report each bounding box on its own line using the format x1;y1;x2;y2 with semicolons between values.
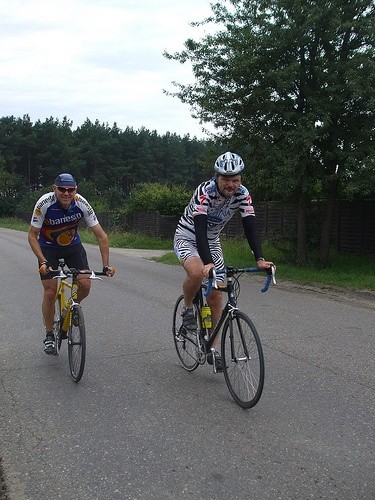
201;303;212;329
60;300;70;331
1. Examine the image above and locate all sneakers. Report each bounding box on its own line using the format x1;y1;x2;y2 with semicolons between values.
207;351;228;369
183;306;197;330
43;334;55;354
72;308;80;326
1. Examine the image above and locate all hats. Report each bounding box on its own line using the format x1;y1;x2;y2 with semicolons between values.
55;173;76;188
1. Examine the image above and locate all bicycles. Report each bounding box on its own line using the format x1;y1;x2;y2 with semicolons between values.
46;259;113;385
171;263;277;410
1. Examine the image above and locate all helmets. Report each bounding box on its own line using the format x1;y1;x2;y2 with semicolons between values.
214;151;244;175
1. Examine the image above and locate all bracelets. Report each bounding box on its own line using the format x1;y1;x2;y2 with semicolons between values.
257;257;265;261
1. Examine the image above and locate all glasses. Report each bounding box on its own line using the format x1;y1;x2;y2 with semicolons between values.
54;186;76;192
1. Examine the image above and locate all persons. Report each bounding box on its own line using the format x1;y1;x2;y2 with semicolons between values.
174;152;276;371
29;174;116;352
